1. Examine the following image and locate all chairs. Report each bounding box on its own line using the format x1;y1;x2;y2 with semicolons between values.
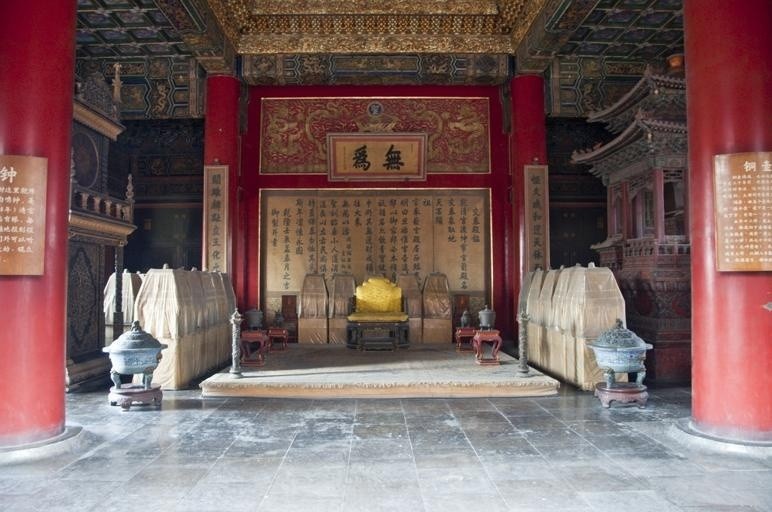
345;273;410;352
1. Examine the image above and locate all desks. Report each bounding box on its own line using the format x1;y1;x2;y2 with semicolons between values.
454;325;503;365
238;327;289;367
